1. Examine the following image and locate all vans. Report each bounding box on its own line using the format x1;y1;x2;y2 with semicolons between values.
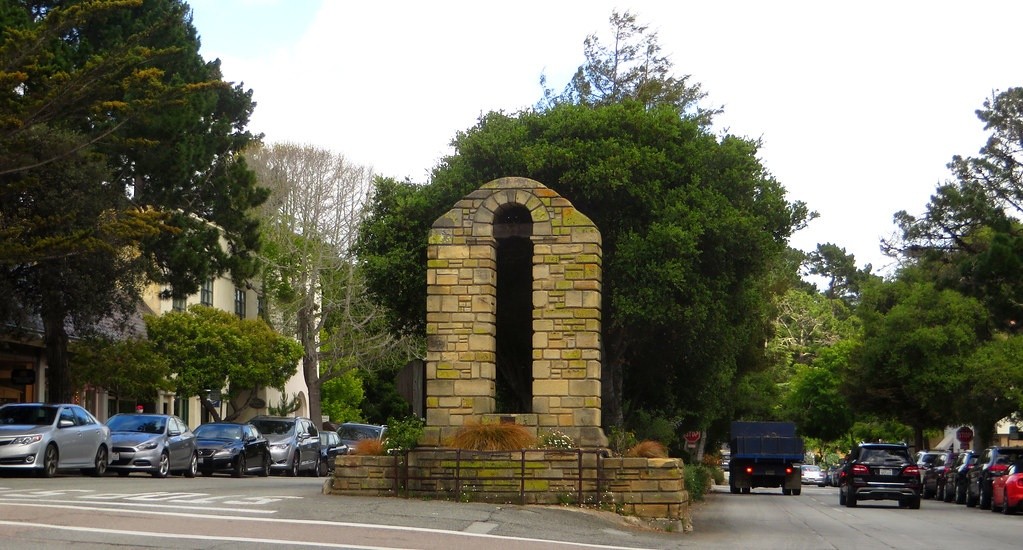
246;415;322;477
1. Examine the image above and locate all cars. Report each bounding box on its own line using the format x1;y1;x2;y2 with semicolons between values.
826;466;839;486
318;432;348;474
193;422;272;477
0;403;114;476
800;464;827;487
721;455;730;470
102;414;200;478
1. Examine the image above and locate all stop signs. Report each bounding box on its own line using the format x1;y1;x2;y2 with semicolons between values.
682;431;701;442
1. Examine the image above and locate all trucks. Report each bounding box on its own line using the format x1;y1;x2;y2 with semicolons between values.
728;421;804;498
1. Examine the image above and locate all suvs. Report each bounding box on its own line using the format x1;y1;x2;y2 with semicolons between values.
943;449;985;504
914;449;949;485
922;451;962;499
336;420;391;456
839;440;922;511
965;446;1023;508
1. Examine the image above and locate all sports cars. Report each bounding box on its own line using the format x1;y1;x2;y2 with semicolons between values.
989;455;1023;515
